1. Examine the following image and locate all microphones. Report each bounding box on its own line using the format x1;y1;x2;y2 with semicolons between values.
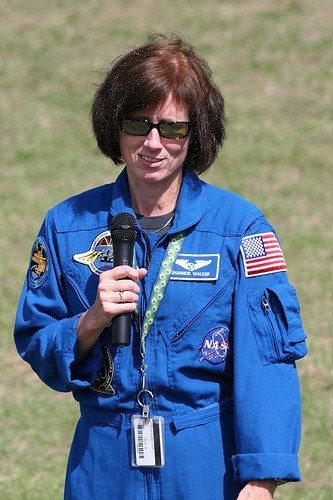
110;212;138;347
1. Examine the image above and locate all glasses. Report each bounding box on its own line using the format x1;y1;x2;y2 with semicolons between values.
117;117;195;140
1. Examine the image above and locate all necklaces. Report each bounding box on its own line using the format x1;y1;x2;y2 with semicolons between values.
152;212;176;234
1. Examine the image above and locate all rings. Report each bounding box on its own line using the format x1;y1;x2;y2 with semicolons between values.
119;290;124;303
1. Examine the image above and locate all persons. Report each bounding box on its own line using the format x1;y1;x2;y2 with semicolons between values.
13;31;307;500
